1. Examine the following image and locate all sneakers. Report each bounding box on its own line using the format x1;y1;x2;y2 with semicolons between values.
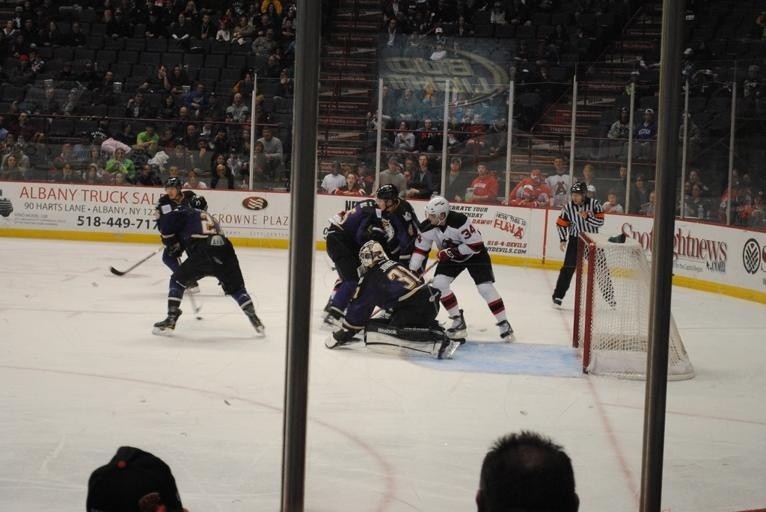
446;309;467;332
155;310;182;329
552;293;561;304
496;317;513;338
244;307;265;333
436;334;455;359
330;306;341;321
188;279;201;289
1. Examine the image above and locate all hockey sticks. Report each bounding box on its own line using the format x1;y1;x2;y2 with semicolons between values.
163;236;204;314
110;245;164;276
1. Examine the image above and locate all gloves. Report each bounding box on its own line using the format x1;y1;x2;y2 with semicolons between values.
333;325;353;343
370;226;382;242
437;246;456;263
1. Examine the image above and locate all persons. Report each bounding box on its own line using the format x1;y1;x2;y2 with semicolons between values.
447;115;506;156
721;168;744;225
210;165;229;190
255;94;266;112
679;113;699;143
395;121;415;154
254;140;264;176
690;170;708;193
333;240;467;359
214;127;231;153
164;166;182;187
166;143;189;164
446;157;470;203
14;111;32;130
409;195;514;343
580;165;605;210
230;126;250;189
138;124;158;157
637;108;657;140
373;157;406;199
159;126;182;144
416;116;441;154
192;139;214;171
408;154;440;198
544;157;578;208
185;122;198;146
0;114;9;131
182;167;208;189
470;163;498;201
365;0;603;115
608;104;636;139
335;172;364;196
736;173;766;228
686;183;712;218
60;161;74;182
631;173;652;217
684;182;694;217
211;155;231;176
213;0;297;91
2;156;24;181
117;121;136;146
55;144;77;182
3;131;27;177
517;185;539;208
476;434;579;512
324;184;399;325
321;163;346;194
152;205;267;337
106;148;136;178
200;117;216;136
612;164;633;213
646;189;657;217
551;182;618;312
83;163;100;187
255;125;282;171
88;144;98;162
225;93;249;123
0;0;213;111
155;178;209;294
601;188;624;213
741;65;761;118
510;168;547;210
377;183;421;267
136;164;154;187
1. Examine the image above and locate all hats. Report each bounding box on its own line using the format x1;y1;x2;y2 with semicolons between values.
435;26;442;33
644;109;654;114
621;106;628;113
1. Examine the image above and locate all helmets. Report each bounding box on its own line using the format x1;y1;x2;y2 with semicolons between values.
357;240;388;267
163;177;184;189
569;181;587;193
424;196;450;222
189;195;208;211
376;184;399;200
